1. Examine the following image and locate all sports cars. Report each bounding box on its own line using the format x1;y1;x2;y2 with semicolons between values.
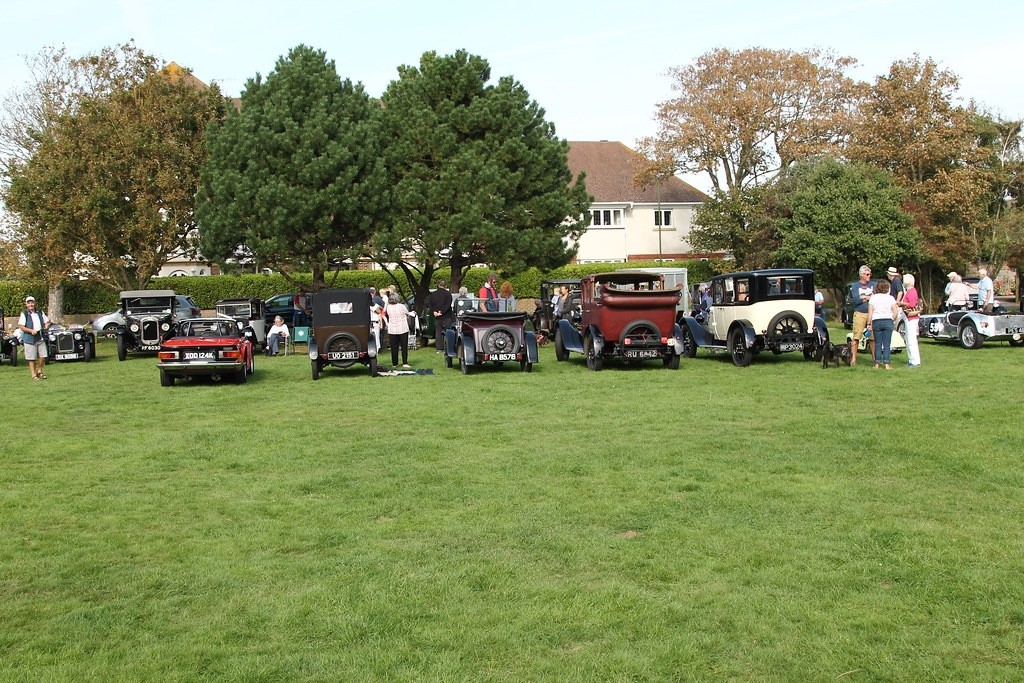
896;299;1024;349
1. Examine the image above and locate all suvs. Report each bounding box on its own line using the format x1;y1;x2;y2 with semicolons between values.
938;277;1008;315
679;268;830;367
309;288;380;380
554;269;685;371
0;305;19;366
440;296;539;375
530;278;583;339
212;297;273;351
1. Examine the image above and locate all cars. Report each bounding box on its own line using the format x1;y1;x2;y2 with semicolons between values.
402;288;479;311
42;319;96;365
841;278;891;329
159;317;254;388
92;295;202;339
265;291;313;333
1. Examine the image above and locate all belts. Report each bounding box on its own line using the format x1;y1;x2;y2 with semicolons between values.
371;321;378;323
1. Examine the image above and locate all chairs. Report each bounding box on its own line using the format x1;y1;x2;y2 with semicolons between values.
216;327;228;336
182;327;194;337
278;326;312;356
994;305;1008;313
725;290;739;303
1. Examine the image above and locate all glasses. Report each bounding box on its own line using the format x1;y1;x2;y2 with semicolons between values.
493;281;497;283
864;273;872;276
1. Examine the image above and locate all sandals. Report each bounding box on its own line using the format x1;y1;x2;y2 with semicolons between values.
32;376;40;381
36;372;47;379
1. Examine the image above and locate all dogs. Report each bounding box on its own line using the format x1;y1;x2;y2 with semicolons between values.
822;344;852;368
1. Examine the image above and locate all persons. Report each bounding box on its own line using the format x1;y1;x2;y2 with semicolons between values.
645;264;970;370
977;268;994;313
19;297;51;380
262;315;290;356
370;271;574;368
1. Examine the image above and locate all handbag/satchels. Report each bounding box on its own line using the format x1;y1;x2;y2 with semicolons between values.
902;297;923;317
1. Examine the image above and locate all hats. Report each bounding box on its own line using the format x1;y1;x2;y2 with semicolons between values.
369;287;376;293
885;267;900;275
947;271;957;277
25;296;35;303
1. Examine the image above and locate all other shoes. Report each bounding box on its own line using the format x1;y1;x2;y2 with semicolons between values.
262;348;269;352
272;352;276;356
908;363;920;368
874;364;880;369
885;363;893;370
